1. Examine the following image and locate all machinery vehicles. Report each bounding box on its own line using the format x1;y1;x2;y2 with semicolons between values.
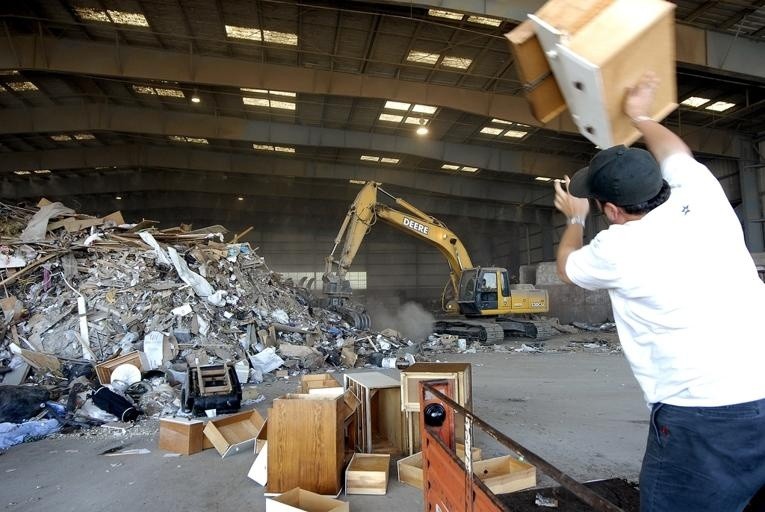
322;179;557;348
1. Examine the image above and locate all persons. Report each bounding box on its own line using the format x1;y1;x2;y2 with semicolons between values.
550;69;765;512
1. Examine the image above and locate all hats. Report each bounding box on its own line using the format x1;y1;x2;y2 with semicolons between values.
567;143;662;206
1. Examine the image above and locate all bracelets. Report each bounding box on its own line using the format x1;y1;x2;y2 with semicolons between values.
631;115;652;131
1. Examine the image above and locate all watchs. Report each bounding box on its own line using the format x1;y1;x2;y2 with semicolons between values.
566;215;586;227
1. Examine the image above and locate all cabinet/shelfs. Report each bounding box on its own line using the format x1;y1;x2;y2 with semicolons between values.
262;360;475;499
